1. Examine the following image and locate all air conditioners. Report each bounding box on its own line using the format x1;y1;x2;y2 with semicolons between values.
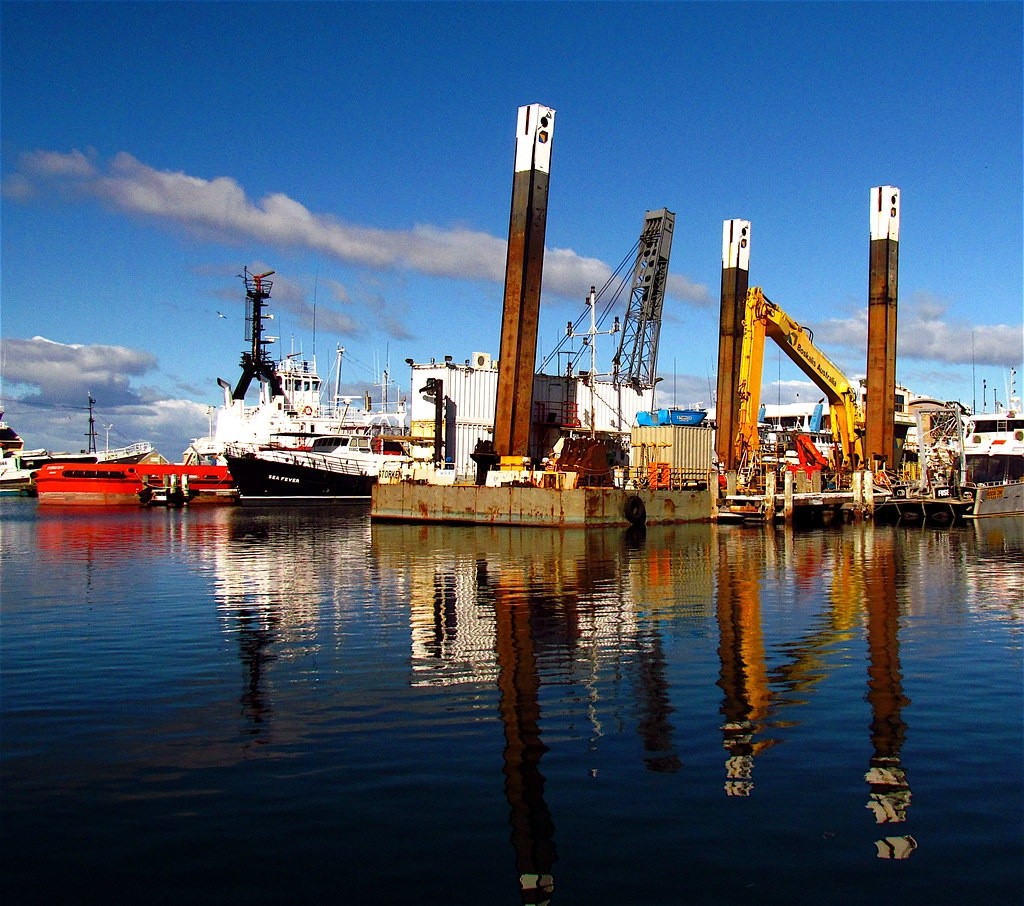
473;352;492;371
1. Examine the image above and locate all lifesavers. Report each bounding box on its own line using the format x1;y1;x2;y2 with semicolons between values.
304;406;312;415
623;495;644;523
877;473;886;483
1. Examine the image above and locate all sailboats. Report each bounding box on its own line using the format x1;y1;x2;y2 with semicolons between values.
633;326;717;426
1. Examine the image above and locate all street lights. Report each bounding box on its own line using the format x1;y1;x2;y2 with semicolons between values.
206;405;216;448
102;424;114;461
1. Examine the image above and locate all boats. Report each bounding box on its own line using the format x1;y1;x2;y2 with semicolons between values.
960;328;1024;489
0;262;412;507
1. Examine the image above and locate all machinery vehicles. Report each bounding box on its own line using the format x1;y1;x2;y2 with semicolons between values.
734;286;928;498
